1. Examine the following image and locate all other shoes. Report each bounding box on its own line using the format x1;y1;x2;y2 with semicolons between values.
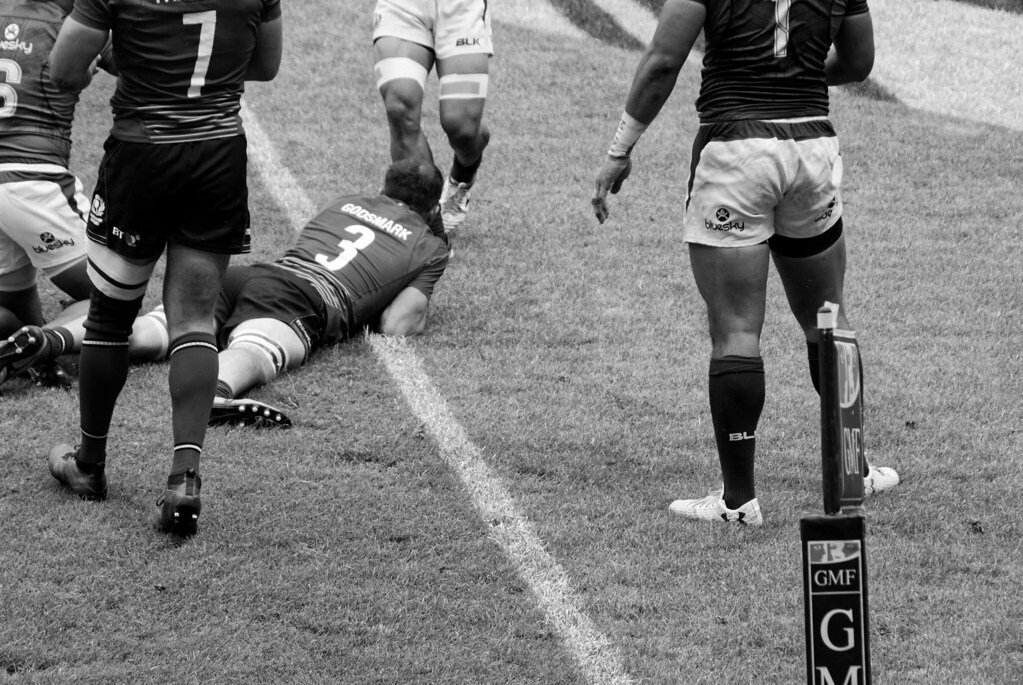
27;359;71;391
208;396;292;429
0;325;47;386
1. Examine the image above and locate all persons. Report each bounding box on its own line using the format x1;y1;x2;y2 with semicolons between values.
49;0;283;537
0;107;451;429
371;0;493;234
0;0;118;389
592;0;901;527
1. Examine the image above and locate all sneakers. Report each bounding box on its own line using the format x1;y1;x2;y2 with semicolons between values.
864;466;900;495
439;175;471;234
669;481;762;525
154;467;202;536
48;443;108;502
